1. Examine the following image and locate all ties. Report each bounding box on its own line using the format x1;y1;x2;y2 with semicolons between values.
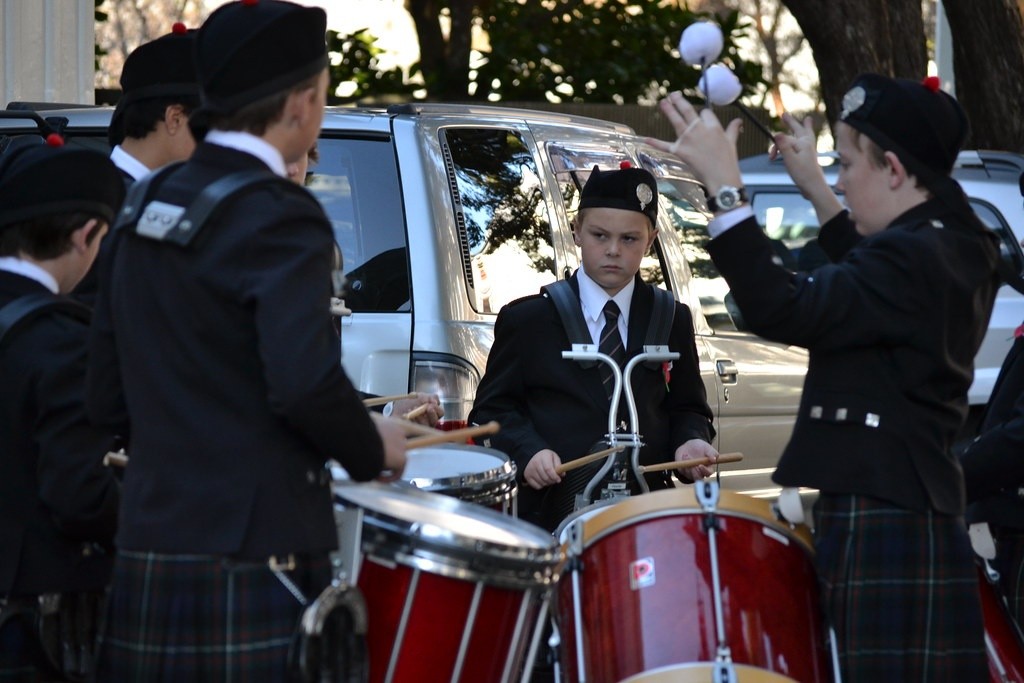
595;300;626;402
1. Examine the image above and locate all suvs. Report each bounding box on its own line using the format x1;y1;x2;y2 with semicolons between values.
734;146;1024;426
0;96;821;527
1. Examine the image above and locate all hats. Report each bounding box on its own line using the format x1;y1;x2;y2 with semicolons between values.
0;134;127;223
578;161;659;225
119;22;201;98
190;0;330;100
836;71;972;185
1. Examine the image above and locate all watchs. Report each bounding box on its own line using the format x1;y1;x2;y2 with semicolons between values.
708;186;747;212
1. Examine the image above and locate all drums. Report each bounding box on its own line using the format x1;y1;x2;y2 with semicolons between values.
544;474;835;683
321;474;562;683
376;438;523;522
971;554;1024;683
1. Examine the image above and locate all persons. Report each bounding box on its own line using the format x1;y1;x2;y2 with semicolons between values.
0;0;445;683
469;168;721;531
646;78;1024;683
